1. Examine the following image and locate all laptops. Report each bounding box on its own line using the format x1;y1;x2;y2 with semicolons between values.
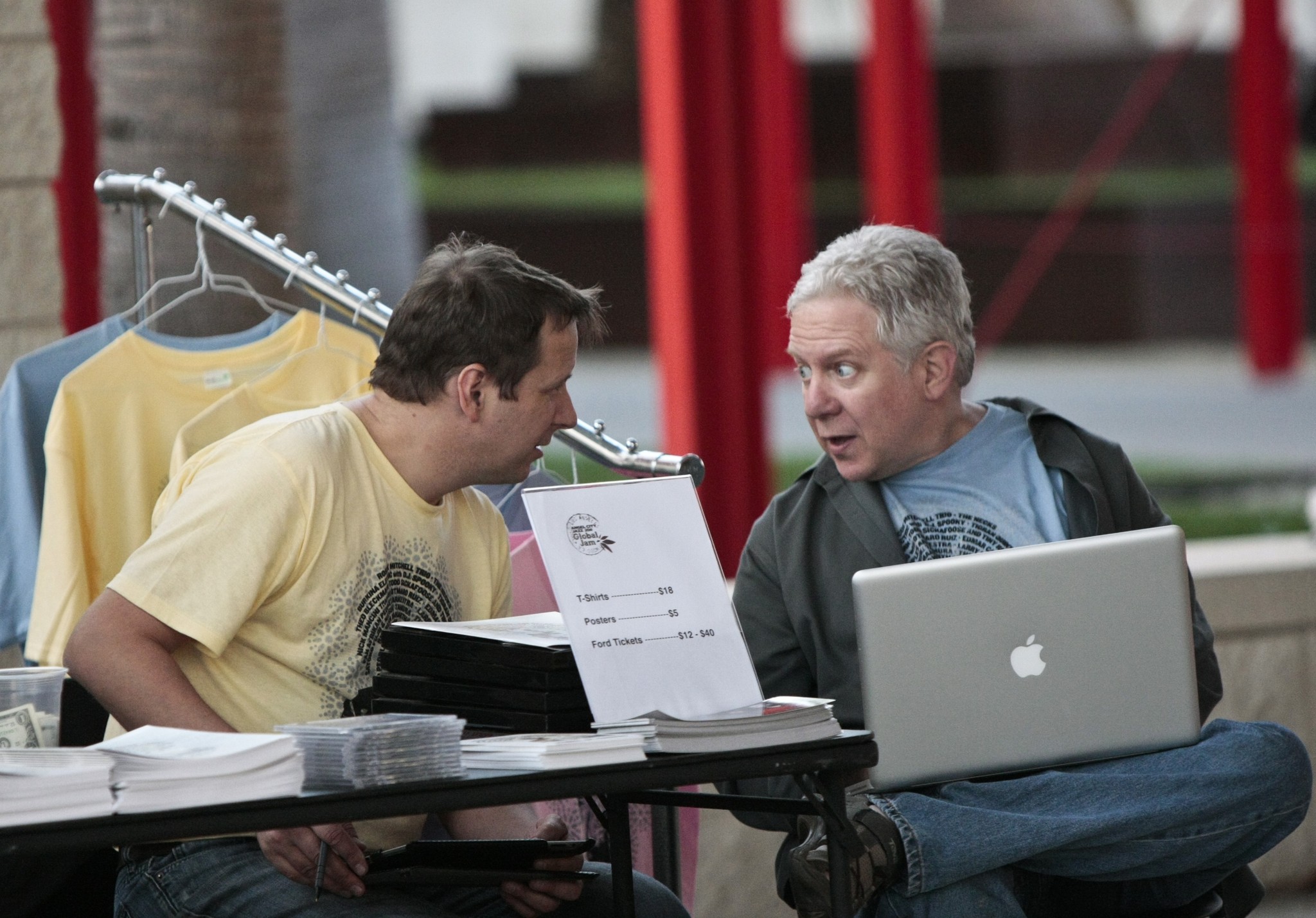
845;524;1202;797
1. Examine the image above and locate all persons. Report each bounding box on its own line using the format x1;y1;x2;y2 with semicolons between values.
60;232;692;918
713;222;1316;918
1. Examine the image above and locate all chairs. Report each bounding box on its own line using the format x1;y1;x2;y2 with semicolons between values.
118;192;578;557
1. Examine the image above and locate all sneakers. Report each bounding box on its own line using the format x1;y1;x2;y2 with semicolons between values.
788;791;906;918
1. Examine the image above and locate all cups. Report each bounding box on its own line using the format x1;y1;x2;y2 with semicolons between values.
0;666;69;749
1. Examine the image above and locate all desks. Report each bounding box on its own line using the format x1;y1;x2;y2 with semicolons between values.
0;728;878;918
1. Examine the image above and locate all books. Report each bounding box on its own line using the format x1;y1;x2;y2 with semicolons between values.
363;613;605;734
462;696;852;776
0;723;305;828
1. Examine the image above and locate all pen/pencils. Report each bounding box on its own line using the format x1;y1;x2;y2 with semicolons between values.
314;840;329;902
